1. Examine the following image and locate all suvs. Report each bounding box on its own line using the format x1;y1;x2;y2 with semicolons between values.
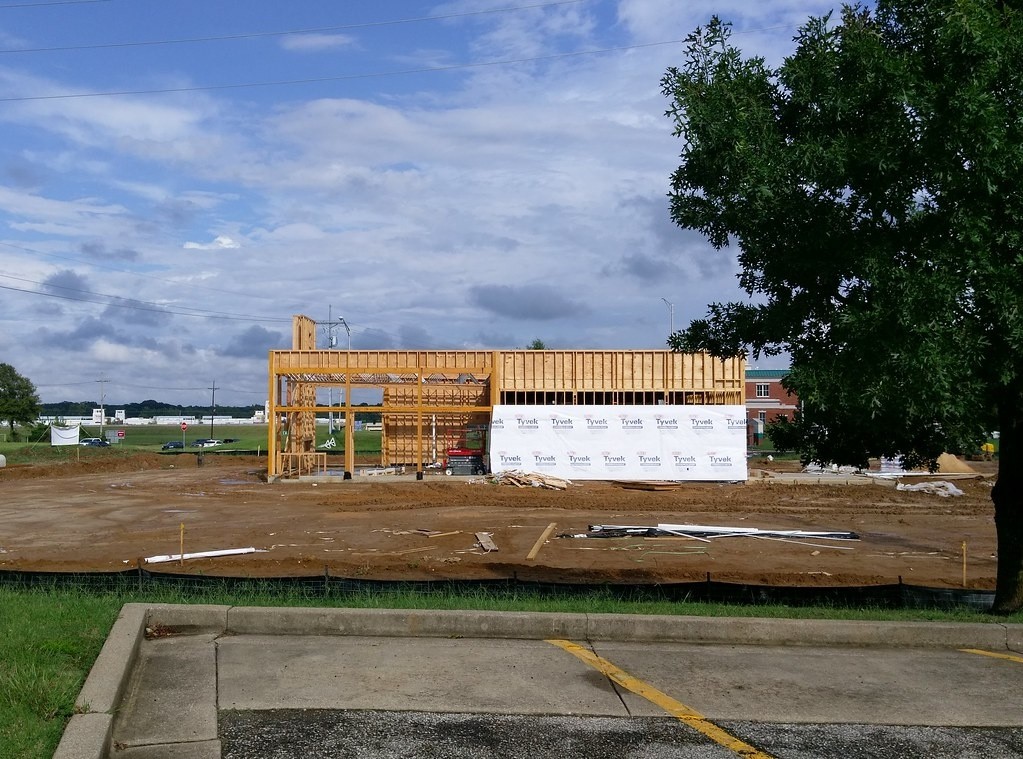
80;437;101;446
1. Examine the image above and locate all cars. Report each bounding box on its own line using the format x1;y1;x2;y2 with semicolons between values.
223;438;240;443
191;438;225;448
89;441;111;448
161;441;184;451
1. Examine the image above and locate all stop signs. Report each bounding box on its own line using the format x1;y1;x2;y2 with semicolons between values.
181;422;188;432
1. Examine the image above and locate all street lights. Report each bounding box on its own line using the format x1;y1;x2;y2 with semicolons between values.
660;296;674;337
336;316;351;349
